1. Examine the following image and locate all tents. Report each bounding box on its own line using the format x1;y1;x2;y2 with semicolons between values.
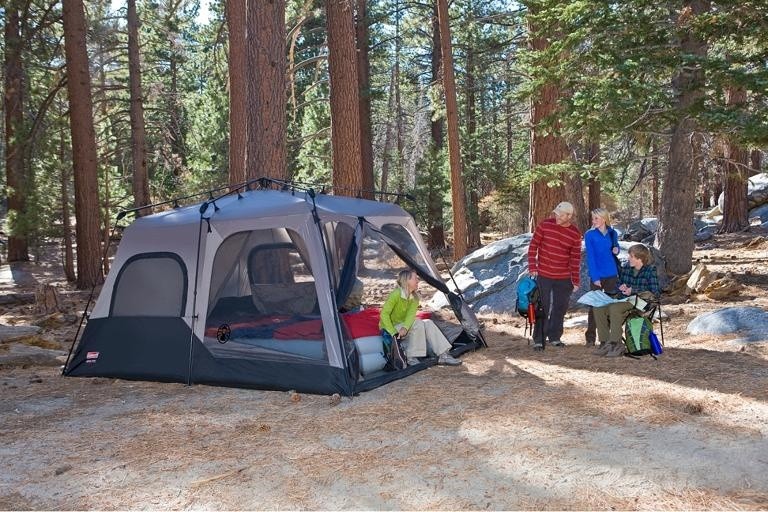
62;175;490;401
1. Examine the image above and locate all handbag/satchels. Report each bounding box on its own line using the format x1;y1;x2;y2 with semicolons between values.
617;261;622;274
379;334;407;371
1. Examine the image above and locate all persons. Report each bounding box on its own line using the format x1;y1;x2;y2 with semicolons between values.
525;200;582;351
378;267;463;368
591;244;662;360
584;208;621;349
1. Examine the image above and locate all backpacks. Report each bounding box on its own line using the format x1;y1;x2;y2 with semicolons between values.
516;274;545;320
625;314;653;355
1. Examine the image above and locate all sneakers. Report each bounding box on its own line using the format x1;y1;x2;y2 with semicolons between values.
592;341;610;355
437;355;462;366
551;340;563;346
586;340;595;346
407;358;421;366
606;342;625;357
533;343;544;351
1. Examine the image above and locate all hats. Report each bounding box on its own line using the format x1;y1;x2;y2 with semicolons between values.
551;201;574;216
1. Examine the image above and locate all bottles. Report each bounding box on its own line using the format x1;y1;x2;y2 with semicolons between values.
528;300;536;323
649;328;662;355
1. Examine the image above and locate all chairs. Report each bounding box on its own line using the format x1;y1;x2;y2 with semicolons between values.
606;296;664;348
